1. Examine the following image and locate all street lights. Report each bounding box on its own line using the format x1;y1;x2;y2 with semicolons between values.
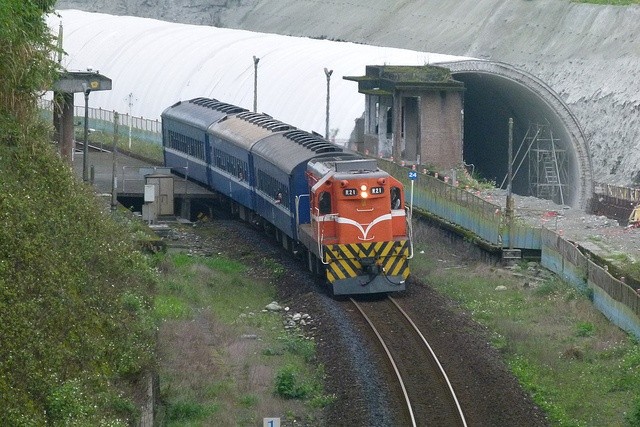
324;67;334;140
252;55;260;112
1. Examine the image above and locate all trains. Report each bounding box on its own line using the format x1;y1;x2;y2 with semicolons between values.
160;97;414;294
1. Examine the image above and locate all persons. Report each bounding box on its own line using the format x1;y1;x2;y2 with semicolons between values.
392;192;401;209
275;192;282;204
239;173;243;182
